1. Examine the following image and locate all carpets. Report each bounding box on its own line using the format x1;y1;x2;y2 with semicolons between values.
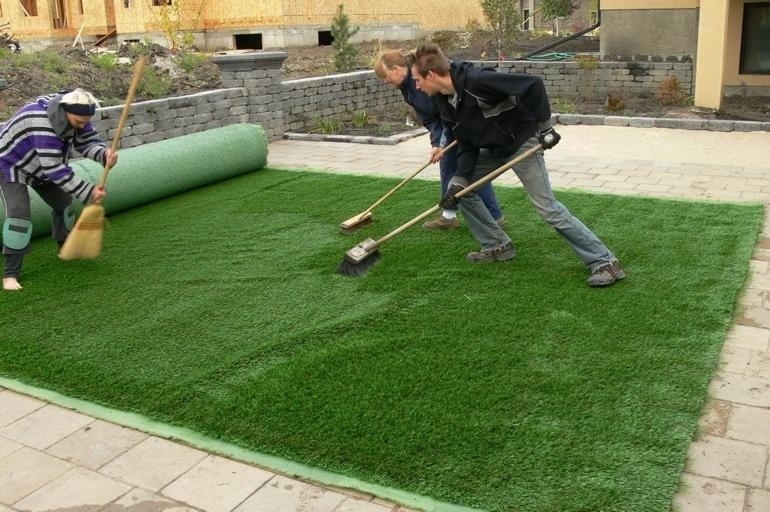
2;163;769;510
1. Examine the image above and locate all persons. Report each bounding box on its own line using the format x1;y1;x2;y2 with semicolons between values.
409;42;627;288
374;49;506;231
0;87;118;291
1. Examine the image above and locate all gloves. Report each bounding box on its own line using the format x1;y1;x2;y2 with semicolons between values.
538;127;562;150
439;184;462;211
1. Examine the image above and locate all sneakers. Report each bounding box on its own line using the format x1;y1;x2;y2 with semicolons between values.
422;215;461;230
496;215;505;228
468;241;517;263
587;260;626;286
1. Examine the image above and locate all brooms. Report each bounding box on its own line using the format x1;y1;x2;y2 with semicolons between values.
338;144;544;278
337;138;462;235
58;56;147;261
